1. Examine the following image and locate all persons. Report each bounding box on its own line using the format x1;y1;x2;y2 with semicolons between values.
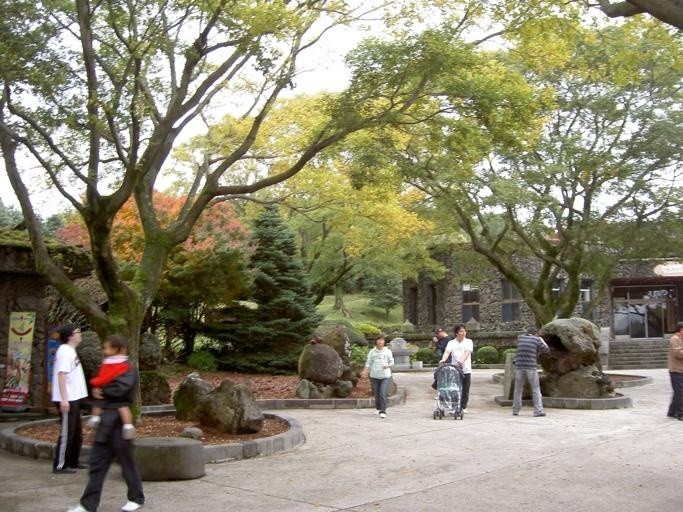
432;324;473;413
512;328;550;417
365;335;394;418
69;333;145;512
51;325;89;474
667;321;683;421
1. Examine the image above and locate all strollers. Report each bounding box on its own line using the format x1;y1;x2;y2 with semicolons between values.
434;363;466;421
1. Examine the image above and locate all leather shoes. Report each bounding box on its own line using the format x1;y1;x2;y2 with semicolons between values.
53;464;87;473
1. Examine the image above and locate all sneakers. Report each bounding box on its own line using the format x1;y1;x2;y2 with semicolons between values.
374;409;386;418
122;424;137;440
68;505;89;512
121;500;144;511
534;411;547;416
87;416;101;429
